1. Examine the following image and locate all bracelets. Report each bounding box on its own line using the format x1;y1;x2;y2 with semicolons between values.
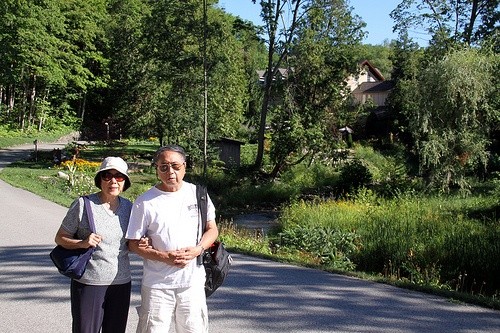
197;244;204;256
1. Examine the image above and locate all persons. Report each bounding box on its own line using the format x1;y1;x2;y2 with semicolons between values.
125;146;218;333
55;156;152;333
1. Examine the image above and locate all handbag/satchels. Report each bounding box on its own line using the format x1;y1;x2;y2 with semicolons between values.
196;184;234;298
49;196;97;280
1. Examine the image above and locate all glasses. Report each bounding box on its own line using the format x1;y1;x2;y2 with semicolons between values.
97;172;126;182
156;161;185;173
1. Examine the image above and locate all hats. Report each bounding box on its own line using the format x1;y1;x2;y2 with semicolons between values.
94;157;131;192
